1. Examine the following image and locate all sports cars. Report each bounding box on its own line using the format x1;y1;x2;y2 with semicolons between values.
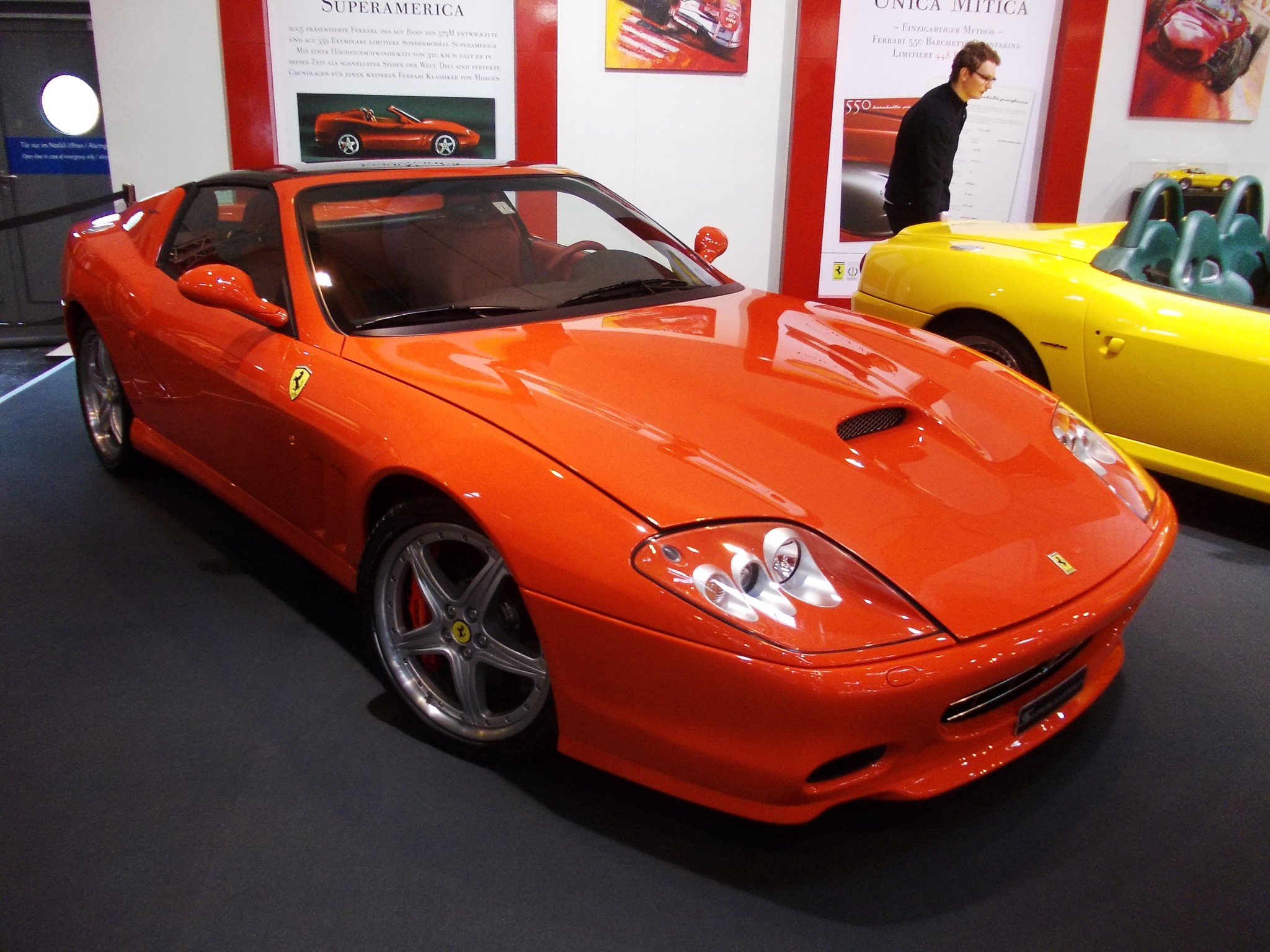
851;176;1270;502
1143;0;1270;95
316;105;482;158
1154;168;1238;191
640;0;742;58
59;162;1178;825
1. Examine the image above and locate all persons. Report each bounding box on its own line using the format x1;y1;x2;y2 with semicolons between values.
880;39;1002;238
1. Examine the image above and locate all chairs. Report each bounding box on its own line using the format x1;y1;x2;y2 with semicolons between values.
358;107;377;122
432;188;533;304
1169;208;1254;305
236;192;353;321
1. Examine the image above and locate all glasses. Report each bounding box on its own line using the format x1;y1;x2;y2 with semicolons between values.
968;67;996;85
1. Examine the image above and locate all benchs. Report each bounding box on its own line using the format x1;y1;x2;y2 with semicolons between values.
1097;175;1264;285
